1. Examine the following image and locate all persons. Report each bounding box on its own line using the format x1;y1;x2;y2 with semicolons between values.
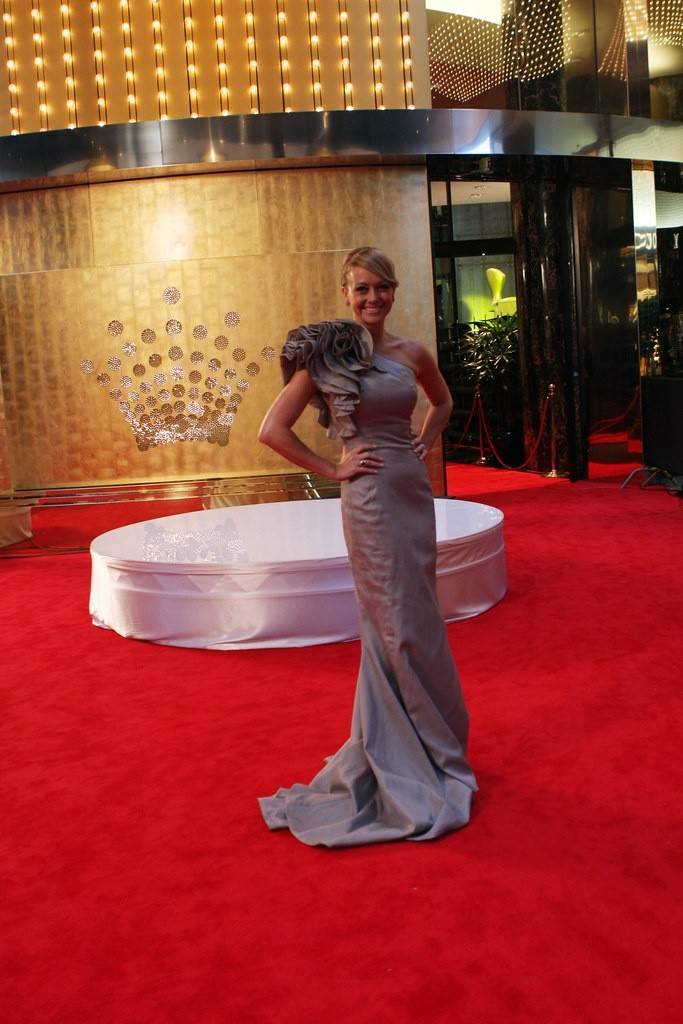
258;246;479;848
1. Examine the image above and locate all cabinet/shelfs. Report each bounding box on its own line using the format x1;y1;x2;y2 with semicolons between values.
641;374;683;475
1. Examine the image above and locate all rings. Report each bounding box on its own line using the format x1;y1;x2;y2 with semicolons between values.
360;458;366;466
